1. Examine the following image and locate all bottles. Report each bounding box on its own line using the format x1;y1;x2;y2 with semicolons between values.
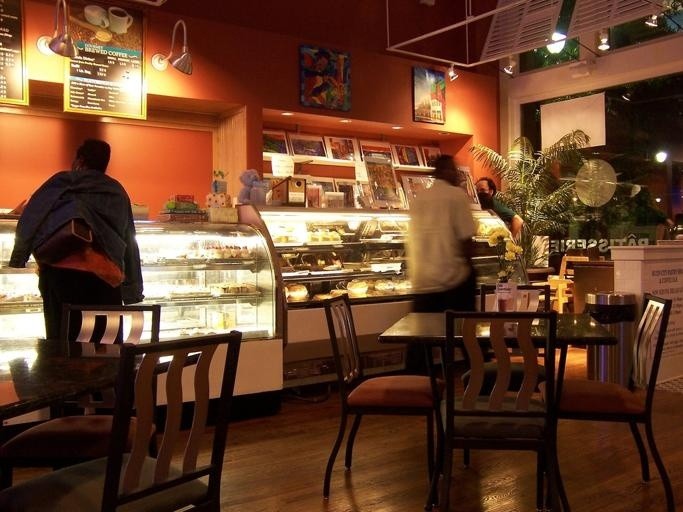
669;225;682;240
249;183;265;205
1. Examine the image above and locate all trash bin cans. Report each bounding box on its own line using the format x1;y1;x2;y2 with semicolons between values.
585;291;636;387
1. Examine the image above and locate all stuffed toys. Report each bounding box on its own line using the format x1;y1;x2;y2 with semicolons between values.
239;168;269;205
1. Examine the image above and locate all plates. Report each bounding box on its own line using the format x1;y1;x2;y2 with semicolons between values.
133;221;158;224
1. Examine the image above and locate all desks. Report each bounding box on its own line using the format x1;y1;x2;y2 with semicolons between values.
0;346;202;491
376;311;617;512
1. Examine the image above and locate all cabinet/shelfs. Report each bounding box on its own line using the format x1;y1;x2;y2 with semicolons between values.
236;202;514;405
258;107;448;206
0;217;285;462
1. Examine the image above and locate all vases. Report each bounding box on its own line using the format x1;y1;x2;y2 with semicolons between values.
493;282;516;312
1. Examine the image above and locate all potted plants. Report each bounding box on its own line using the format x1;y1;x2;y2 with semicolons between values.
467;130;625;282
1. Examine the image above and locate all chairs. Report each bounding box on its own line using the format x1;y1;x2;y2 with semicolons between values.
0;302;161;492
441;310;559;512
460;283;551;468
548;256;590;315
322;292;446;509
549;292;675;508
0;330;243;512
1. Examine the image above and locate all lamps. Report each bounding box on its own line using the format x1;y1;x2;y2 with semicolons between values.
447;63;459;82
150;20;194;76
36;0;80;59
597;29;611;51
645;13;658;28
504;55;518;75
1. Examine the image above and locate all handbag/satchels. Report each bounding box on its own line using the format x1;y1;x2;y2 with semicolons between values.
32;219;93;264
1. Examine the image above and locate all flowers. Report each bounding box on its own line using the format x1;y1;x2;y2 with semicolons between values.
486;226;523;284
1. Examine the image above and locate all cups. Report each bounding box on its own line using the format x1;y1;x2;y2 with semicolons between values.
305;186;319;208
130;205;150;220
107;7;132;34
83;6;109;29
324;192;343;208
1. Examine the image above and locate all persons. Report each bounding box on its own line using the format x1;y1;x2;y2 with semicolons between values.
8;140;145;471
406;155;491;375
478;193;497;216
475;178;524;239
634;191;674;225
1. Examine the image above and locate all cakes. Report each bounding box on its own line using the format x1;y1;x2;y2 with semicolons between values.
218;283;257;293
205;245;254;261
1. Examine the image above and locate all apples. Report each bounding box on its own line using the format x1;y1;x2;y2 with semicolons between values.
166;200;176;209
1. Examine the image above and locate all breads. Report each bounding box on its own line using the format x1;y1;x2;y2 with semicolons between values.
285;280;413;301
270;231;341;243
282;252;345;270
